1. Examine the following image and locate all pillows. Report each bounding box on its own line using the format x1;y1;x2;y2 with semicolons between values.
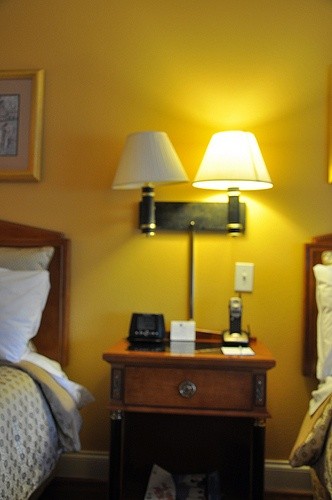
313;263;331;382
0;246;55;365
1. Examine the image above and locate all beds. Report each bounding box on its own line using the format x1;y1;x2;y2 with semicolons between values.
0;220;98;500
289;235;332;500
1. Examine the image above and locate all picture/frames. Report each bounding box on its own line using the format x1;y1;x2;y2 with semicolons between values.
0;69;45;183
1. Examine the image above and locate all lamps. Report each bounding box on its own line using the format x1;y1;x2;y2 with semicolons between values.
197;131;272;238
112;132;188;237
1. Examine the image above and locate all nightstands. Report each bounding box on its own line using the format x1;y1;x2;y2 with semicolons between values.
102;337;276;499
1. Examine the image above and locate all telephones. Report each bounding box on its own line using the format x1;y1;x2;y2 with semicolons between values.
221;297;249;346
